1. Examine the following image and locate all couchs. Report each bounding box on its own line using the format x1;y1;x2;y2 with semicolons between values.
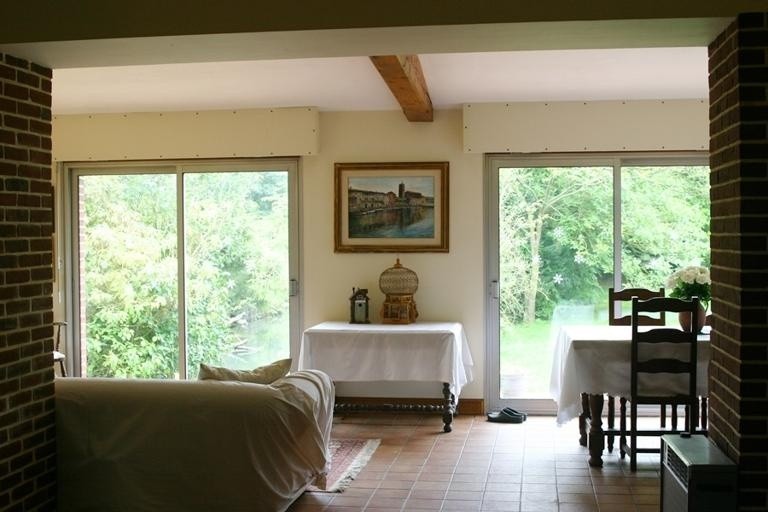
54;369;336;512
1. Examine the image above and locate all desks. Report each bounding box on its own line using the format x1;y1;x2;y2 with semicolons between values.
297;322;473;433
549;325;710;468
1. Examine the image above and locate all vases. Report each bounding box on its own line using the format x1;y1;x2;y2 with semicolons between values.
679;298;706;334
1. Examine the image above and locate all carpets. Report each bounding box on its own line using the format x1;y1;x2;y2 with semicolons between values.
305;438;383;492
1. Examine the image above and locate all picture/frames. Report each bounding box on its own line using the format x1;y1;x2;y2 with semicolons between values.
333;163;449;253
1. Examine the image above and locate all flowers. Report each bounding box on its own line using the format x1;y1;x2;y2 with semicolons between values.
668;266;712;312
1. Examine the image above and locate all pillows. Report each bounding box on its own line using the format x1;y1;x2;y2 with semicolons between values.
198;358;292;384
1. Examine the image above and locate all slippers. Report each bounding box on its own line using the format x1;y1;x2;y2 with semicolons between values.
492;407;526;421
488;408;524;423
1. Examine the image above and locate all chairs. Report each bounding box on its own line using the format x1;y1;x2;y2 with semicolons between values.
54;321;68;377
608;288;677;453
620;296;698;473
